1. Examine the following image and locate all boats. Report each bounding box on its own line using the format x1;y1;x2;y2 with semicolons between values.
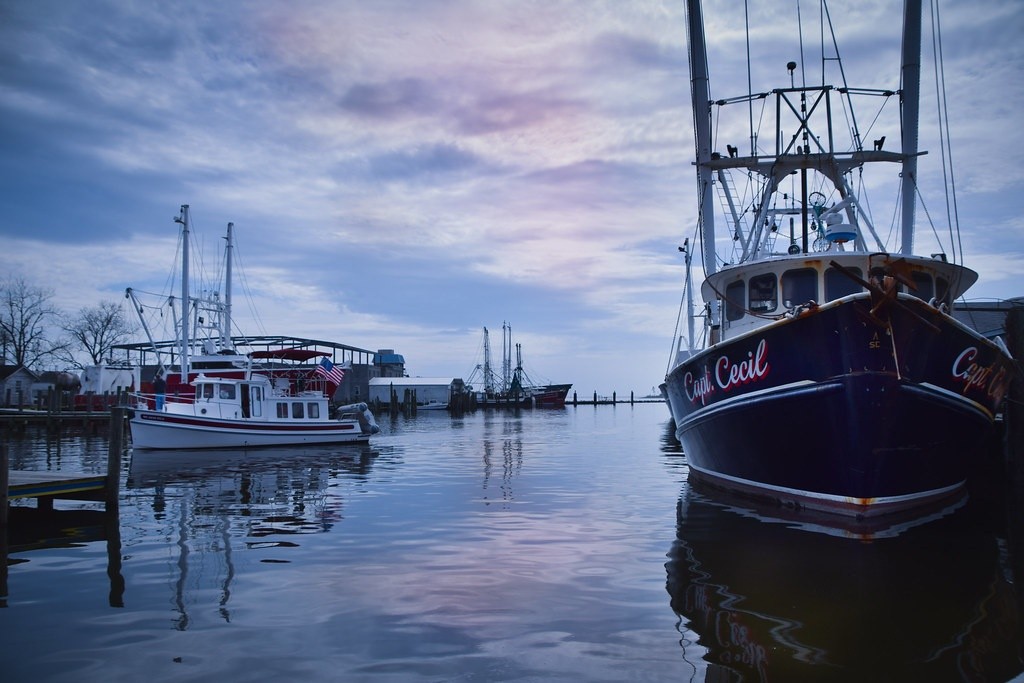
663;472;1024;683
658;1;1016;545
57;203;343;414
125;443;377;514
417;399;449;409
121;368;378;451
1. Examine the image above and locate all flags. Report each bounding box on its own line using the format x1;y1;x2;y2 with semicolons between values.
316;356;344;386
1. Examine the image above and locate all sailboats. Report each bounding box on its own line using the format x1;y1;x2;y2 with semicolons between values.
468;320;573;407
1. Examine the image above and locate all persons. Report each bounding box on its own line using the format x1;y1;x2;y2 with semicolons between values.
153;375;167;411
221;384;230;399
